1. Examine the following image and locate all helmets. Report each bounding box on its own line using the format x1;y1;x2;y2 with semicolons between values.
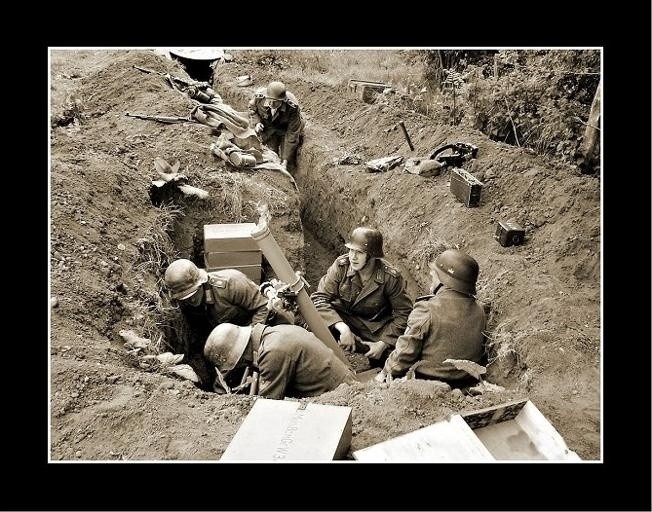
203;323;251;371
267;82;285;100
344;227;384;258
434;249;479;296
165;259;207;299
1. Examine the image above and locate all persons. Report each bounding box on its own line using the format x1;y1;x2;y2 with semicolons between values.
374;249;487;388
202;322;361;399
307;225;413;362
246;81;304;169
164;258;296;394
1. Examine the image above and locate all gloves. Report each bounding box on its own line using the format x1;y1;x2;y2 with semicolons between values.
256;123;264;133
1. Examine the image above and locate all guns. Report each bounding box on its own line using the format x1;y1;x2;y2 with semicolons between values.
125;113;197;125
133;65;189;86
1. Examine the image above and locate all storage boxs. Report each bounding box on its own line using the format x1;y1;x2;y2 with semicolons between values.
203;223;261;285
353;397;584;478
218;399;354;463
